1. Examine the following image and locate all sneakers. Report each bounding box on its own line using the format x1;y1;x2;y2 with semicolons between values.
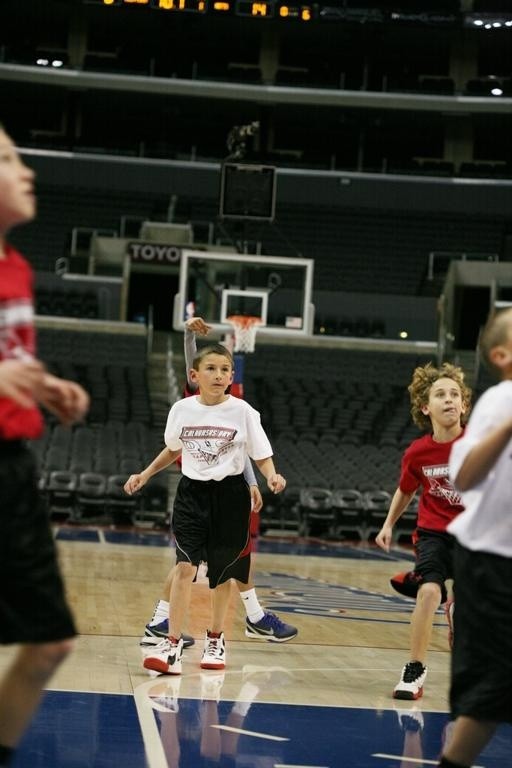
199;672;227;707
392;659;428;701
199;628;229;670
244;610;298;644
141;617;196;648
142;635;185;676
241;664;296;694
149;678;184;717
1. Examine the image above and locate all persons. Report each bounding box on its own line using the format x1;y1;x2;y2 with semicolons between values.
139;317;299;647
137;675;222;767
175;663;285;767
439;305;512;767
121;344;286;675
374;360;473;699
0;129;92;765
394;705;455;766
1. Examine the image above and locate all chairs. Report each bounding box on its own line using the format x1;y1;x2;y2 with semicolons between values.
23;286;477;547
